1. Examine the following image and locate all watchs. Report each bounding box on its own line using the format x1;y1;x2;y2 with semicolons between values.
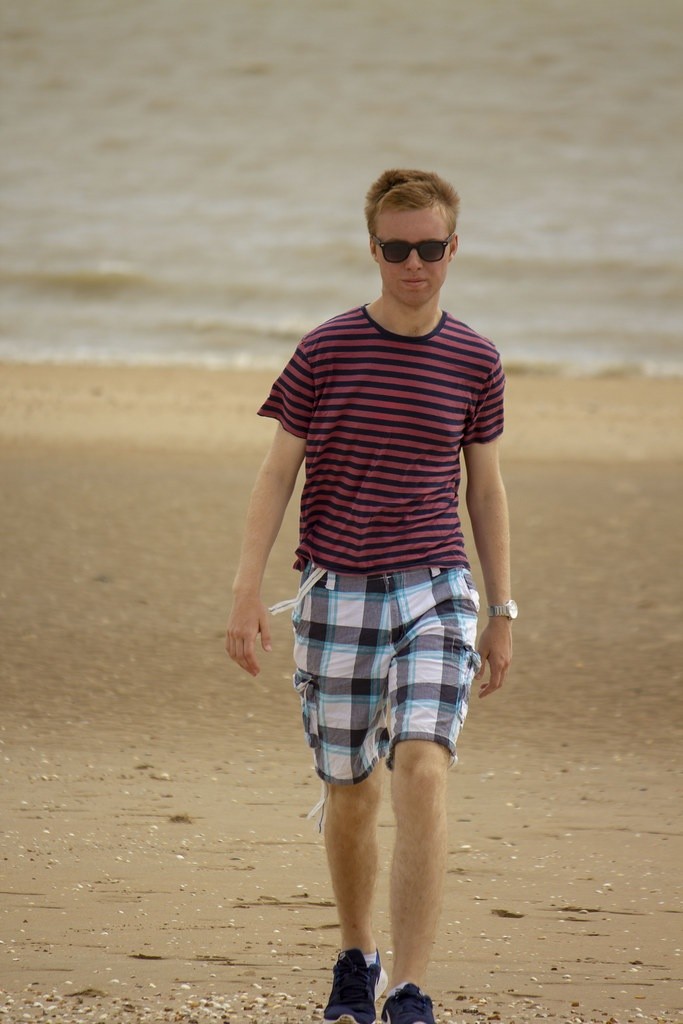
487;600;518;621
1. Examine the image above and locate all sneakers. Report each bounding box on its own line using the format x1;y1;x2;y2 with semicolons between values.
323;945;388;1024
381;981;435;1024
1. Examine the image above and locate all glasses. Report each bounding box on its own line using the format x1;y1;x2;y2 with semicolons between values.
371;232;456;263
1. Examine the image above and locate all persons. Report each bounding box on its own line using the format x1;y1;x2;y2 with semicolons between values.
222;167;514;1024
